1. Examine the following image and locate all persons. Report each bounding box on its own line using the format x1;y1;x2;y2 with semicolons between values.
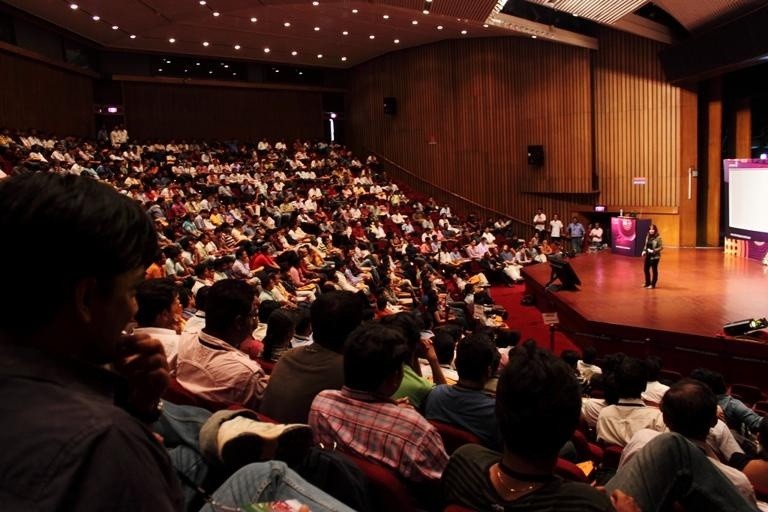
2;174;768;512
0;123;376;183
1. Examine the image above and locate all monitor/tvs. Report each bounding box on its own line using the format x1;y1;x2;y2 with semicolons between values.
383;96;397;116
527;145;543;164
549;257;581;286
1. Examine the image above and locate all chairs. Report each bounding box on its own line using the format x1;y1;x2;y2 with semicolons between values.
0;176;768;512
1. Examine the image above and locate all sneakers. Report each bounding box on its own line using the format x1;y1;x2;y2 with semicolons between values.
217;418;314;464
